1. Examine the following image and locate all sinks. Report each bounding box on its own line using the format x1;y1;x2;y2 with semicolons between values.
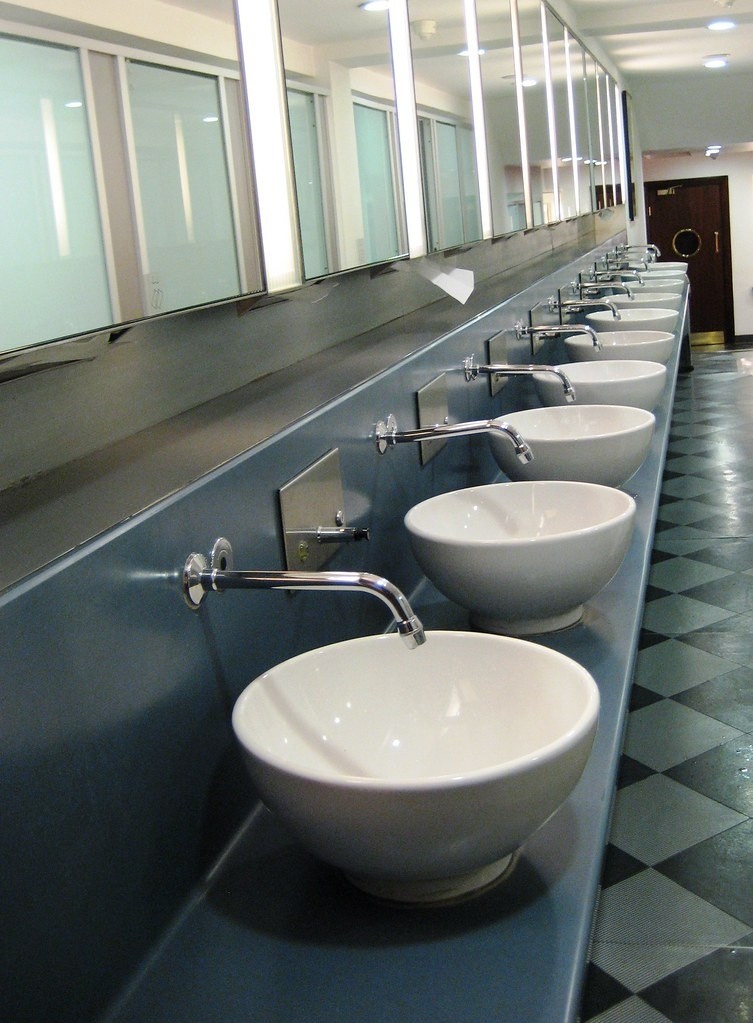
621;278;684;295
584;307;680;334
599;292;683;313
630;261;689;273
626;269;686;282
531;359;667;418
402;480;638;623
486;404;657;497
564;330;675;369
231;630;601;903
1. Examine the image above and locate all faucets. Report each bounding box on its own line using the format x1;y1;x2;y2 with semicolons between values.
600;256;649;273
462;353;577;404
588;267;644;286
612;248;654;264
515;318;603;352
620;242;662;257
375;420;534;465
570;280;634;301
182;551;425;650
548;294;620;321
